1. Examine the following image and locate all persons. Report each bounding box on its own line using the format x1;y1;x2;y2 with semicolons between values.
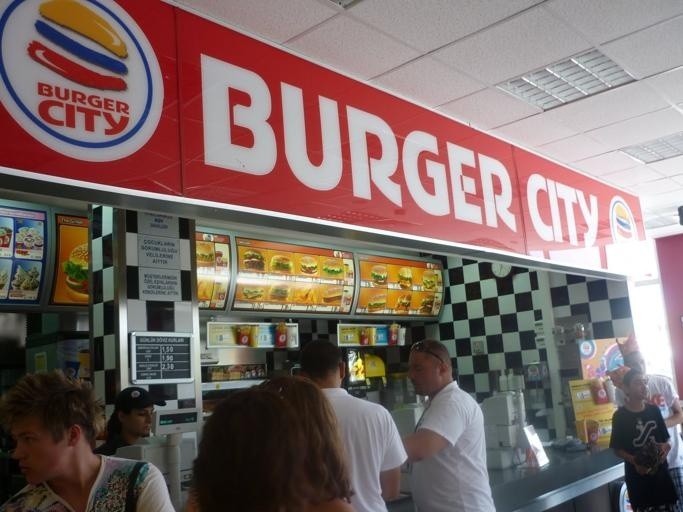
94;387;166;456
609;369;678;512
186;390;301;512
251;376;356;512
615;351;683;512
300;339;408;512
402;338;497;512
0;368;177;512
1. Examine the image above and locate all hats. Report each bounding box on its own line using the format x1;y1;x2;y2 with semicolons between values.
116;387;166;409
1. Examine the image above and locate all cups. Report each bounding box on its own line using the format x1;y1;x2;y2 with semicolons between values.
361;327;376;345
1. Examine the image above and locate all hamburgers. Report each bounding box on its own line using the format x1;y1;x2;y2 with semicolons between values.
367;265;437;314
243;250;265;270
615;203;634;238
270;285;289;301
300;256;317;275
60;243;90;303
243;284;264;301
196;244;215;266
324;287;341;304
323;259;343;276
270;255;292;274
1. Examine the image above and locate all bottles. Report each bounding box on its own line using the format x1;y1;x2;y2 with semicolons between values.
250;325;259;348
498;368;513;391
604;377;615;402
389;321;406;346
575;320;584;347
275;319;289;346
342;327;360;345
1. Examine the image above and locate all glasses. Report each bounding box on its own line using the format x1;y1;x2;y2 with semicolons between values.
411;341;444;364
